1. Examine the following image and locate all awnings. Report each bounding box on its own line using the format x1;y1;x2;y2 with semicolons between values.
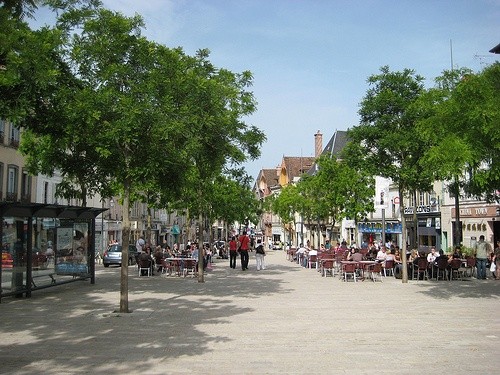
0;200;110;219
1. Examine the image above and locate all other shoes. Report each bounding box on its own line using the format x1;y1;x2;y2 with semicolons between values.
237;245;242;252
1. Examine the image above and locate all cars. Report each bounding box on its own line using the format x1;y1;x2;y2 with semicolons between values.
103;244;141;267
213;241;226;249
272;241;284;250
22;250;48;269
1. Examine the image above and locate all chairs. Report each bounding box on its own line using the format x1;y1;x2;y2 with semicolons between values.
286;247;479;283
137;248;210;279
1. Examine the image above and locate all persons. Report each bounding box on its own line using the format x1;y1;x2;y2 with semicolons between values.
294;243;306;262
303;246;317;268
110;239;117;244
377;244;426;280
237;231;253;271
255;239;266;271
211;236;228;259
361;240;397;261
229;236;240;269
351;242;360;250
341;238;347;249
427;246;465;279
347;247;363;276
334;242;342;254
249;234;256;252
321;240;333;251
492;240;500;280
474;235;491;279
137;234;210;276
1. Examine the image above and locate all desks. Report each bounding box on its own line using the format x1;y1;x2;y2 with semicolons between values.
339;260;358;281
320;259;337;276
359;260;378;281
164;258;197;278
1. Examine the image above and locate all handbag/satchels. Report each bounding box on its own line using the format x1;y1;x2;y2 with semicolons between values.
489;262;496;271
